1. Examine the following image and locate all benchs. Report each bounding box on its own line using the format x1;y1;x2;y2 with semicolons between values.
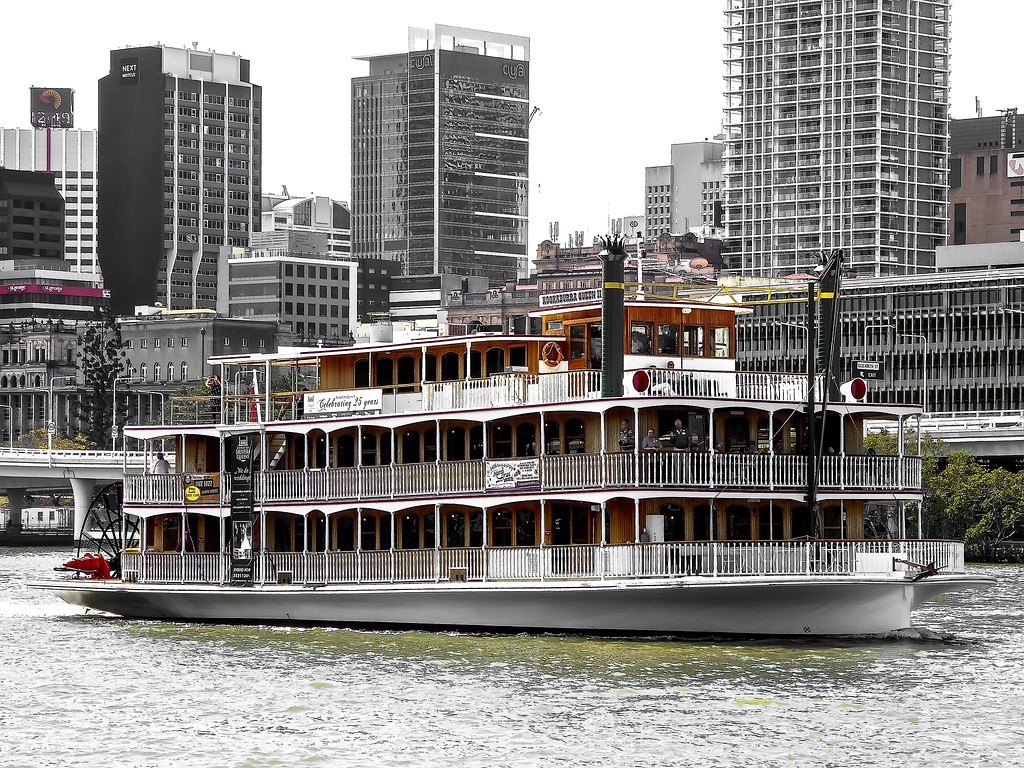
647;375;728;397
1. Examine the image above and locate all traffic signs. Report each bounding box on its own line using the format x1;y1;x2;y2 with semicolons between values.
851;360;885;381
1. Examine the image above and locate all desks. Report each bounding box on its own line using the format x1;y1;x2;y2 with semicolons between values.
825;548;850;571
791;462;807;485
733;462;754;484
733;547;760;572
776;549;798;573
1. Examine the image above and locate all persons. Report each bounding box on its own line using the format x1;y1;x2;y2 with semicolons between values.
175;531;196;552
150;453;170;475
205;376;224;424
773;436;876;486
296;387;311;420
588;330;693;484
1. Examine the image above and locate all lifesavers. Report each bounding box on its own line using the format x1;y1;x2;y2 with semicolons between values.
542;341;563;367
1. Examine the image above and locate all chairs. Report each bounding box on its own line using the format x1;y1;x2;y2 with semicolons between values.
763;552;778;573
718;546;735;573
800;542;825;573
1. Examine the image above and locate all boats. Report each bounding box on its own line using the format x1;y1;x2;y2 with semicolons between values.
0;521;75;547
26;223;975;640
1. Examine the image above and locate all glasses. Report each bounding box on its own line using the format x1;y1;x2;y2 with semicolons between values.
669;364;674;366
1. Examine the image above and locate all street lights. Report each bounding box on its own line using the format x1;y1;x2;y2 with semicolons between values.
769;319;809;401
899;333;926;414
112;376;144;454
234;370;261;425
48;375;75;468
133;389;165;456
864;324;896;362
0;404;14;450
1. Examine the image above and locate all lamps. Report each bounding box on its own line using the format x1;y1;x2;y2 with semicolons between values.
839;378;870;402
